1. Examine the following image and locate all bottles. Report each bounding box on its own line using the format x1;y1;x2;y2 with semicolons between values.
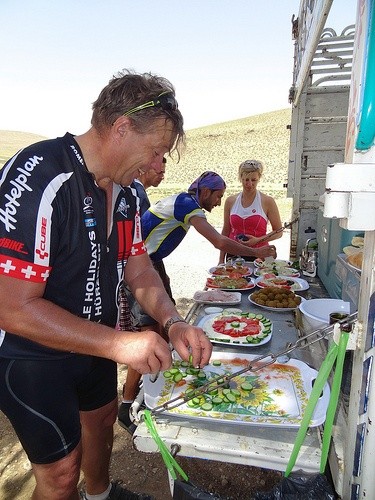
305;227;316;250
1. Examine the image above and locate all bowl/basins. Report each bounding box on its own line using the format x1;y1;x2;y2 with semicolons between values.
299;298;351;340
205;307;223;314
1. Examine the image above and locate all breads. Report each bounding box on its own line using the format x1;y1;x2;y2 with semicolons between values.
346;251;363;269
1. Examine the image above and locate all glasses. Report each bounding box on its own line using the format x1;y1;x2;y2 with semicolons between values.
112;91;178;126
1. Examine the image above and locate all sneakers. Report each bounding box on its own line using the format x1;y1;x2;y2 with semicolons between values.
117;402;137;435
80;479;152;500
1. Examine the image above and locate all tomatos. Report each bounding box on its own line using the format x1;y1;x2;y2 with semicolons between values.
228;272;254;288
213;316;261;337
258;281;291;289
205;272;222;288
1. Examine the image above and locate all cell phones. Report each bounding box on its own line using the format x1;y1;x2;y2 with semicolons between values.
237;234;248;241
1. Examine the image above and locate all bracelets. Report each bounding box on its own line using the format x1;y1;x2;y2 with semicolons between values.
165;316;186;338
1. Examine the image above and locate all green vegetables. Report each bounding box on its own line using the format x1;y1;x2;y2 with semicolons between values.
256;262;279;276
214;276;235;289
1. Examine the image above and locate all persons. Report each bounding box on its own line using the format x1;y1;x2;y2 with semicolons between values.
217;160;283;267
117;172;276;436
115;156;167;284
0;73;211;500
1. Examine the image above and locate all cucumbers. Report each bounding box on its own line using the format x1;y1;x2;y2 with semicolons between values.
208;337;231;342
163;355;253;411
221;311;272;343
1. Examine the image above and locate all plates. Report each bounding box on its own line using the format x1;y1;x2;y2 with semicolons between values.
208;266;253;276
205;276;256;290
248;292;307;312
197;313;273;347
337;253;361;272
225;308;243;312
254;267;300;278
193;290;242;305
255;276;310;291
254;258;293;268
